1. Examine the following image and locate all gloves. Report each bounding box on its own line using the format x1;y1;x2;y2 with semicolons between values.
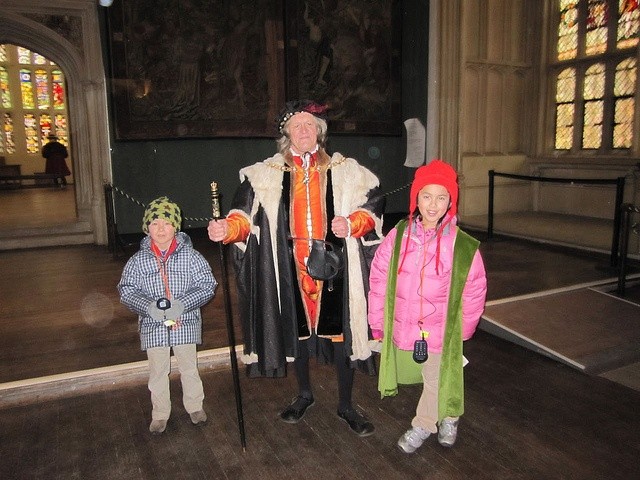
165;299;184;320
148;300;165;322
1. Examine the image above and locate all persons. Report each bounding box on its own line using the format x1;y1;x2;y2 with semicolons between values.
207;100;386;437
367;159;486;453
117;197;218;435
42;134;69;191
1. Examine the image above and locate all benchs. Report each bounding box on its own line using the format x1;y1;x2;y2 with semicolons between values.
0;174;62;191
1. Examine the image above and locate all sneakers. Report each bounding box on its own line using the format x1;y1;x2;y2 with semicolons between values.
437;416;460;447
397;426;431;454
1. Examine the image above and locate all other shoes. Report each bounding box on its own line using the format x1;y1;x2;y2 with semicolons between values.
335;406;376;437
149;420;167;434
280;394;315;423
189;408;207;425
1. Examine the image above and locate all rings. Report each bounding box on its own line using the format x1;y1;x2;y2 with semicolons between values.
335;232;338;235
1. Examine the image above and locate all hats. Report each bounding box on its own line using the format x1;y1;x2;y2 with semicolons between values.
277;99;327;134
47;133;58;139
410;160;458;220
141;196;182;235
